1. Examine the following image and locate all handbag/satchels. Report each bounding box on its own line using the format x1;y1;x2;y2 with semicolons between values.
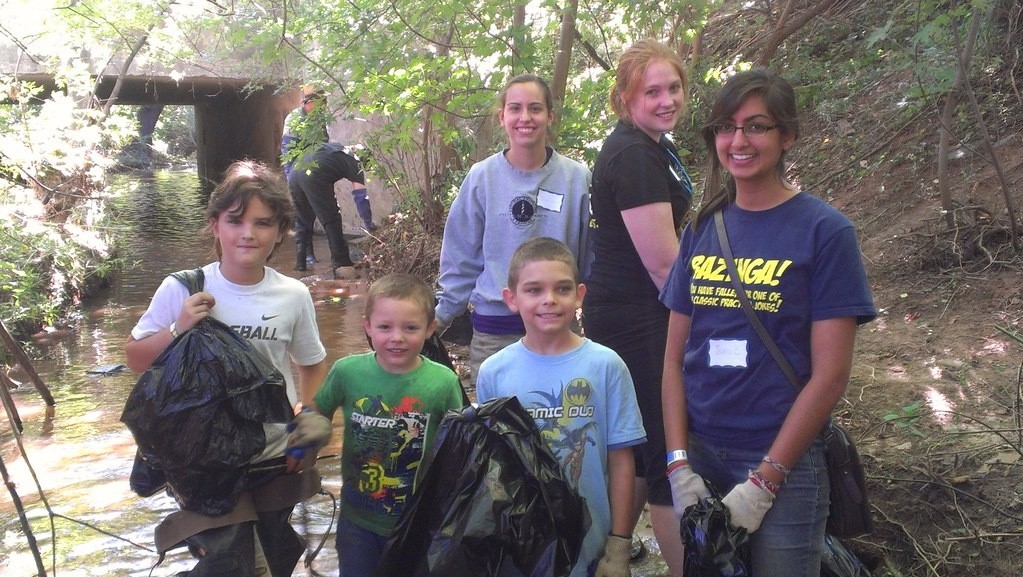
823;425;873;540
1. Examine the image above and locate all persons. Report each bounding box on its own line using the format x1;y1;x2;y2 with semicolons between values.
661;69;877;577
288;143;378;279
127;159;330;577
282;83;328;264
435;74;594;385
310;271;463;577
582;38;695;577
475;236;647;577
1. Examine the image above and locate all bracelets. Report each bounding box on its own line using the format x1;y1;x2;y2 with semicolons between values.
665;450;688;466
761;455;789;476
170;323;178;339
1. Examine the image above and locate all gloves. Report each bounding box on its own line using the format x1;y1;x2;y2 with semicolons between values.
352;189;372;233
720;472;777;534
595;533;632;576
434;315;451;339
667;463;712;519
284;409;333;459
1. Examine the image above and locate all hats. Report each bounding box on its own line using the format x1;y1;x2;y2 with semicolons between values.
301;81;331;103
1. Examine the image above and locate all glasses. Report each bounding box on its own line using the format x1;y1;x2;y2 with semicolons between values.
713;121;781;137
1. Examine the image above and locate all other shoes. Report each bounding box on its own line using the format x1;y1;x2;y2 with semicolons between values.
306;256;317;264
